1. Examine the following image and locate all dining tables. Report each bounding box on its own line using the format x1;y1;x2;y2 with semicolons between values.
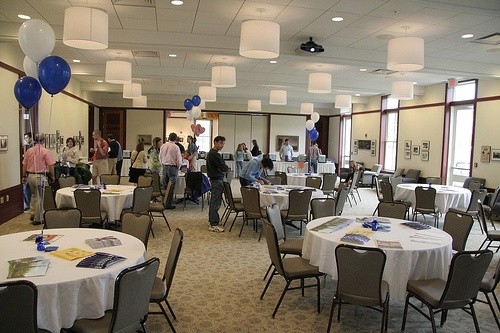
287;173;323;189
120;159;335;178
240;185;323;211
394;184;472;214
302;216;453;300
55;185;137;221
0;228;145;333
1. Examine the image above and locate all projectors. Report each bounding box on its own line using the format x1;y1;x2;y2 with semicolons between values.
294;43;325;56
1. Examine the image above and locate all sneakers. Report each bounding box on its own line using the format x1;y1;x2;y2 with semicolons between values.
208;221;224;227
208;225;225;232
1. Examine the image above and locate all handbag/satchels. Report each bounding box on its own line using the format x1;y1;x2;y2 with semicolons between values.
129;167;132;178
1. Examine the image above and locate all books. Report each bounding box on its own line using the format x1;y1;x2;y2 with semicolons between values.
402;221;431;231
7;256;50;278
309;213;391;234
50;236;126;269
23;234;64;242
102;188;128;195
341;228;404;250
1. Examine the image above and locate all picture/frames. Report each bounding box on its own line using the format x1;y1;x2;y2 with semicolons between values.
0;134;9;151
275;136;299;152
45;130;84;153
352;139;376;156
480;145;500;163
137;134;152;145
403;140;430;161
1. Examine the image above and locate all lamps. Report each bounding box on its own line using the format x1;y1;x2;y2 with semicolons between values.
63;7;108;50
106;60;147;108
239;20;280;59
199;66;413;114
387;37;424;71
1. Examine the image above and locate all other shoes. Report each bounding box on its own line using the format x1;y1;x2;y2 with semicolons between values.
30;217;34;221
33;220;44;225
24;208;30;211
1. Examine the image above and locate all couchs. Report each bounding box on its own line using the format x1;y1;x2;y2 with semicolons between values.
463;177;486;192
402;169;421;184
360;164;382;188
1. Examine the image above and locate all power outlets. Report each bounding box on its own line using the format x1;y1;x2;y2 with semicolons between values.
0;195;9;204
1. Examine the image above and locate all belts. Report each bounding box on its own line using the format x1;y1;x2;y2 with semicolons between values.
29;172;44;174
163;165;175;167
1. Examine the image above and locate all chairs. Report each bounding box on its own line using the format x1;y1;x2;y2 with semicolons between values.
62;256;160;333
149;228;184;333
327;244;389;333
373;177;500;256
263;203;303;280
38;174;174;250
401;250;493;333
0;280;51;333
441;259;500;331
184;165;363;242
260;219;325;318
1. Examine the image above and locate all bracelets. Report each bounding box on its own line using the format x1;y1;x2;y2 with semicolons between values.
22;176;26;178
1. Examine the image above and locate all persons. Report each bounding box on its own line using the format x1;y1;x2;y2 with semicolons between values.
239;157;273;187
22;132;55;225
206;136;228;232
307;142;322;173
279;139;294;161
343;161;366;186
61;137;80;167
234;139;261;177
20;132;38;212
91;128;198;210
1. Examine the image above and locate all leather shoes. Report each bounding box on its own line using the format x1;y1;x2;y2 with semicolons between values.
165;206;176;209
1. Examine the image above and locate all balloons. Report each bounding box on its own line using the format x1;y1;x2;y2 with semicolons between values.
13;19;71;109
165;111;171;117
191;124;206;137
184;95;202;121
205;113;218;120
305;112;320;142
199;113;204;119
